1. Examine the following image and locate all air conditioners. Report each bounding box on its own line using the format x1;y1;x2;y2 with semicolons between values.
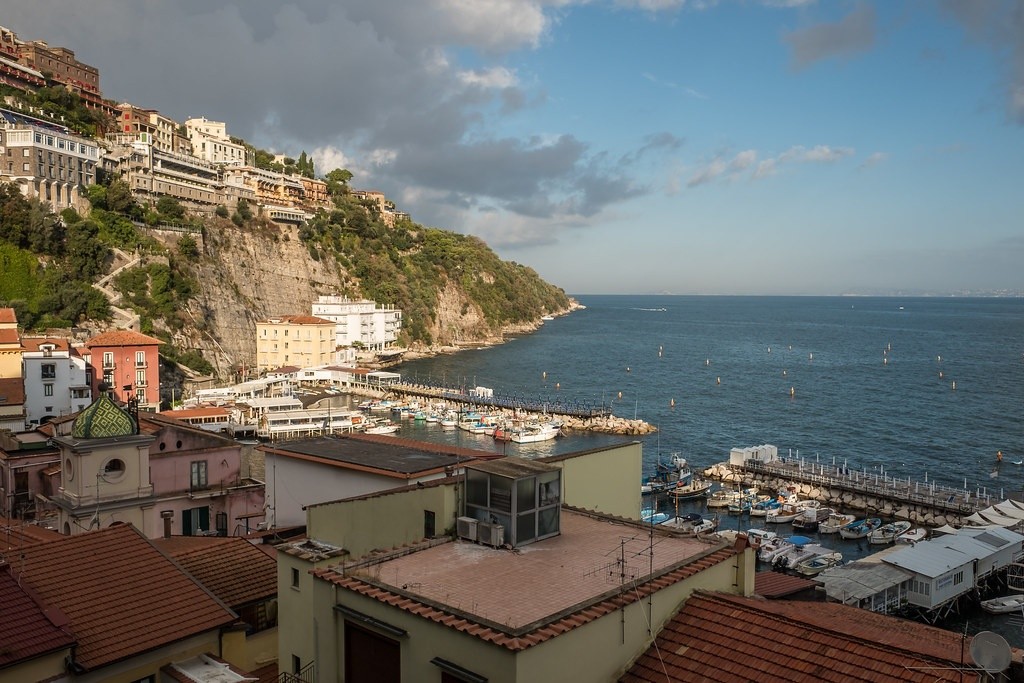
478;521;505;550
457;516;479;544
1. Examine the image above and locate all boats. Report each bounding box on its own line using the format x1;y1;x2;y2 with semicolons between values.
642;415;928;575
979;593;1024;615
171;378;565;444
540;314;556;321
